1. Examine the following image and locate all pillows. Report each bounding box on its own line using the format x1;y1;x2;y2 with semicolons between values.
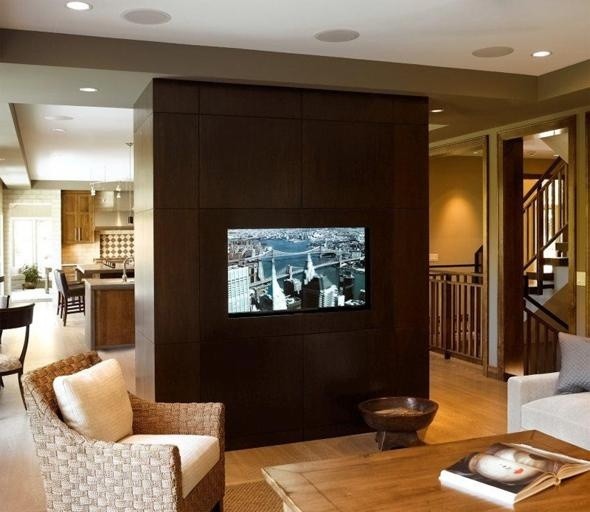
51;357;136;444
554;329;590;397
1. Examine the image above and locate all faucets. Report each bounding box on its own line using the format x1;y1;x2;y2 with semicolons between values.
122;256;134;282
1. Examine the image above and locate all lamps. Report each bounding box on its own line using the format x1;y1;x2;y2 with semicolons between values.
90;142;135;204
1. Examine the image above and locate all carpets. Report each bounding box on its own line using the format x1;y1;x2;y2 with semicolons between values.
222;477;283;511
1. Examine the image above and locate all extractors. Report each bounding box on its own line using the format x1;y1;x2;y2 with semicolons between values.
94;190;134;230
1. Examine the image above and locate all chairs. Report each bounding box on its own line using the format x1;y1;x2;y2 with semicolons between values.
504;368;590;450
1;293;13;311
53;268;83;318
19;347;231;512
57;271;85;327
1;302;38;411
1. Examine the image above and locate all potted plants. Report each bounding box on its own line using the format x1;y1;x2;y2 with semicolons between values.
18;263;42;291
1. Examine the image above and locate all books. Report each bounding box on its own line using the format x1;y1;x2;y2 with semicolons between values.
435;440;590;511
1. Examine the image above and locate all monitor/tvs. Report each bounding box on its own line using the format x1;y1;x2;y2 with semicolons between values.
226;227;371;318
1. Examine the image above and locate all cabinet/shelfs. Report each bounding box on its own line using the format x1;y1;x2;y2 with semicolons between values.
62;266;77;282
62;189;95;245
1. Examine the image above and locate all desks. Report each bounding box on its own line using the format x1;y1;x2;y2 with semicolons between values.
75;261;134;278
261;428;590;511
